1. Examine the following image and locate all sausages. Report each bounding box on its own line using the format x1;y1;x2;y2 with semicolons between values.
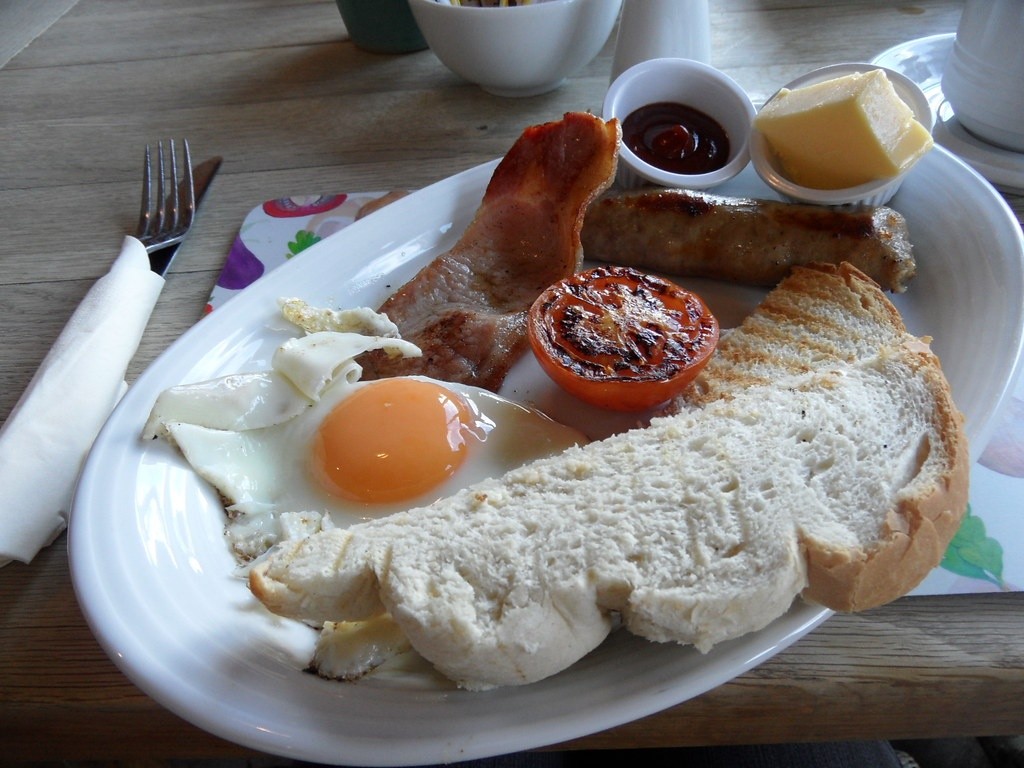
577;189;917;298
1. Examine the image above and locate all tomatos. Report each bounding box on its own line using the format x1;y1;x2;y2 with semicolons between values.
526;265;721;414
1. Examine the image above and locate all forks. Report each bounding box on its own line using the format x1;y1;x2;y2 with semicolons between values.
132;135;195;255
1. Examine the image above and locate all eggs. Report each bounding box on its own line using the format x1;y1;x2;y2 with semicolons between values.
139;294;591;682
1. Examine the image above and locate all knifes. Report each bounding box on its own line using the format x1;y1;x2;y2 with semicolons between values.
140;154;226;277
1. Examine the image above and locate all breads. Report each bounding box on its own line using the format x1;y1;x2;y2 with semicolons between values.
244;258;974;686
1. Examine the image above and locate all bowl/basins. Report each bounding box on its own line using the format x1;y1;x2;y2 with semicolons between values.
411;0;625;97
602;55;757;197
749;60;932;212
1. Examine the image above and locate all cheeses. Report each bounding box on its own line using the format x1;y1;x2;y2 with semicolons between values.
756;68;933;189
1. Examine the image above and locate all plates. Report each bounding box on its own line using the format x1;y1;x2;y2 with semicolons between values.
873;34;1022;205
70;77;1023;768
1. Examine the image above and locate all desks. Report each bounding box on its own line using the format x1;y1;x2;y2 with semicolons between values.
1;3;1023;768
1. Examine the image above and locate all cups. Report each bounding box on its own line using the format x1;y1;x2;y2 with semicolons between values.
942;0;1024;151
337;0;436;56
